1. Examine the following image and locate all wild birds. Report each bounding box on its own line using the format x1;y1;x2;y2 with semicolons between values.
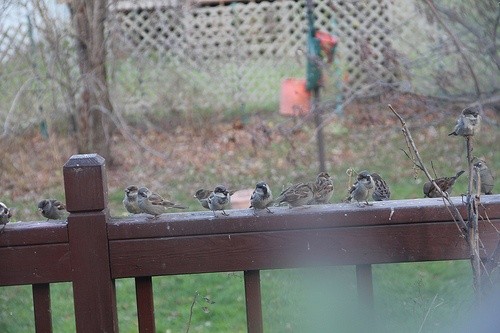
194;189;235;212
138;187;190;219
248;182;273;216
0;202;13;232
38;199;67;221
447;103;482;137
345;171;375;206
315;172;334;204
369;173;390;200
204;186;230;217
275;183;317;210
473;160;496;195
122;186;144;215
424;170;465;198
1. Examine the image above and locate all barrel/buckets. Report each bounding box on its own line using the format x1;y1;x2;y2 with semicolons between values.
278;76;311;116
